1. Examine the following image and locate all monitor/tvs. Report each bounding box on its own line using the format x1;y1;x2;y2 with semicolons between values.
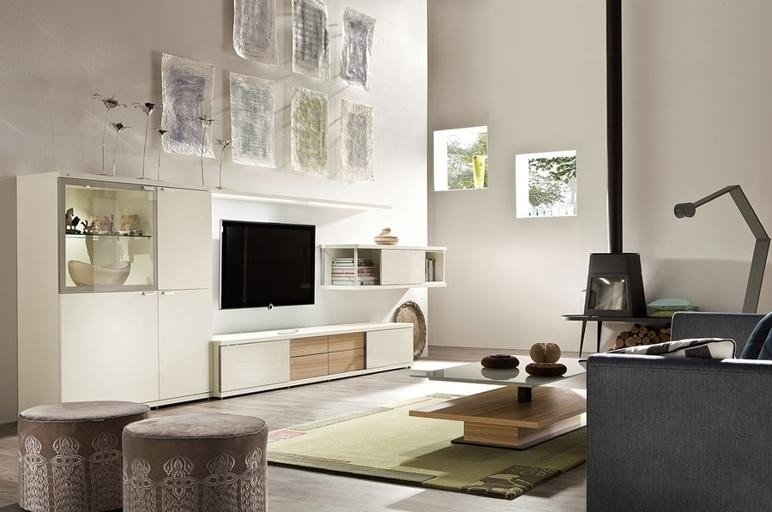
219;218;315;310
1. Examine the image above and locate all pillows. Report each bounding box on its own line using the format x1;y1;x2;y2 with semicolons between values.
579;338;737;371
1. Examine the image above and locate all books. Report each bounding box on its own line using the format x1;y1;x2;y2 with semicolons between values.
426;259;435;282
331;257;377;286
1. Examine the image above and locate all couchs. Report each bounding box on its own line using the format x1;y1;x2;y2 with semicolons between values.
584;312;772;512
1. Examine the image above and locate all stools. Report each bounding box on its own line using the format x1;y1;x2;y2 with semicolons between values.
121;411;268;511
16;400;150;511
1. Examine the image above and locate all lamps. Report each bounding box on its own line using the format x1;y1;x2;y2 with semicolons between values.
673;184;771;312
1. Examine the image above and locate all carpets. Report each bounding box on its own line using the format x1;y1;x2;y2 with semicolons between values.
267;391;585;500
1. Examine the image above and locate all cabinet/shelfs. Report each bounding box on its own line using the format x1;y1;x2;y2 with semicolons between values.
320;242;448;289
210;321;416;398
14;172;213;413
